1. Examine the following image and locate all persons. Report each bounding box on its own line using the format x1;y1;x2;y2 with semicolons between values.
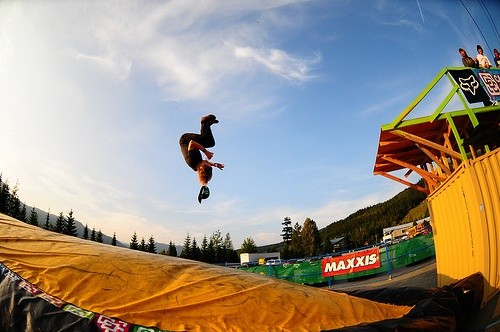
494;48;500;69
179;114;225;203
474;44;492;69
458;48;496;108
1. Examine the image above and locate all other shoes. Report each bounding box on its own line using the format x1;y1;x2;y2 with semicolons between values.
201;115;218;126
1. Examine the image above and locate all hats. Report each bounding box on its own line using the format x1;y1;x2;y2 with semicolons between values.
198;186;210;203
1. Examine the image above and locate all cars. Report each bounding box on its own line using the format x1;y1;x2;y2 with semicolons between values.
382;235;408;246
242;257;322;267
